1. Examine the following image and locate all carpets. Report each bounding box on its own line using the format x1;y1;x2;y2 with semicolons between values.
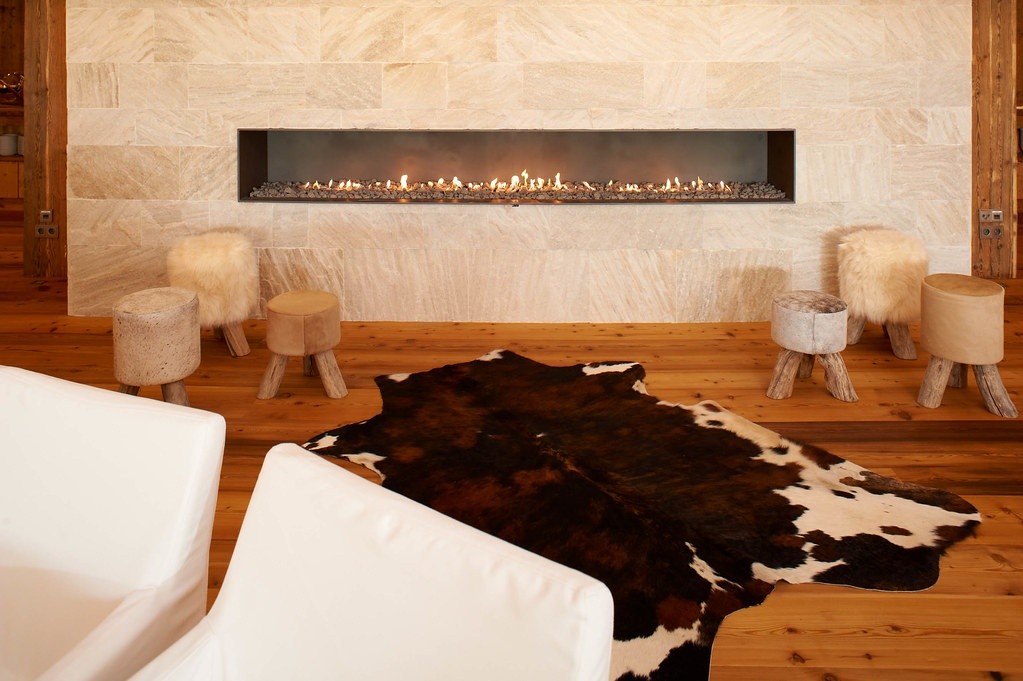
284;348;984;681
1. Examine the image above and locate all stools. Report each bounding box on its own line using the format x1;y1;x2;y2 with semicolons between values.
920;273;1020;419
766;288;859;402
257;290;347;400
836;232;926;361
166;228;260;358
113;286;201;409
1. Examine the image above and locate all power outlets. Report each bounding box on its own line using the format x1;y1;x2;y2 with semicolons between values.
980;210;1004;238
33;211;60;239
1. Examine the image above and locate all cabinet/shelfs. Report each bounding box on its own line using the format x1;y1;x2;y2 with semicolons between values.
1;106;26;199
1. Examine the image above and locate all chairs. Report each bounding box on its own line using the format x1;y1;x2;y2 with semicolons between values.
1;362;226;680
133;443;613;679
